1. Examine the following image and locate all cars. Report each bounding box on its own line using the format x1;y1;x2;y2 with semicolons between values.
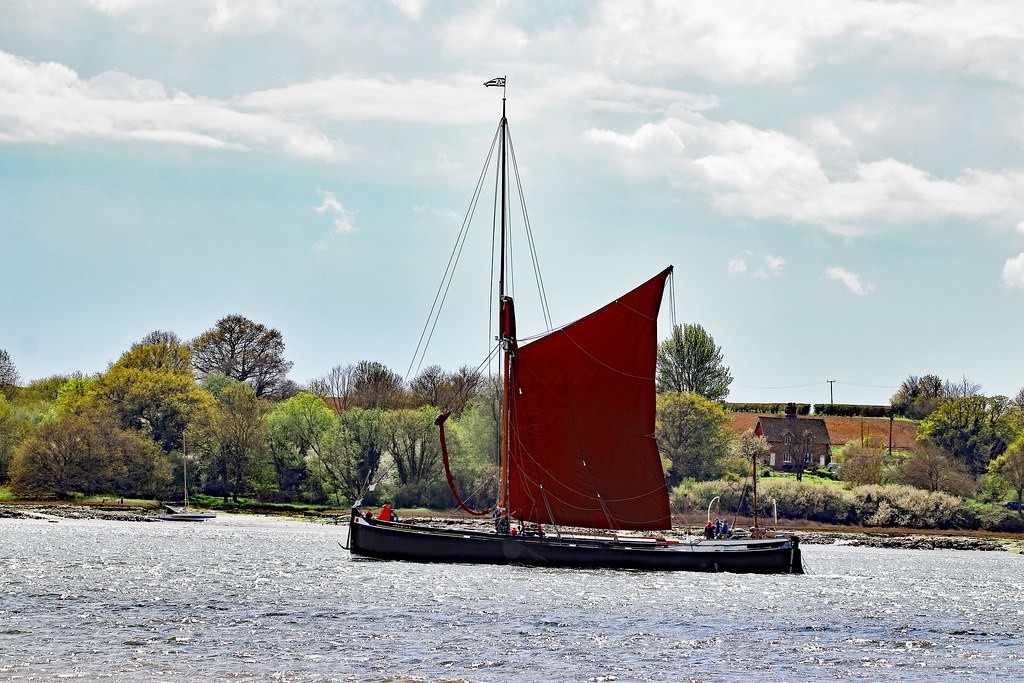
819;462;841;481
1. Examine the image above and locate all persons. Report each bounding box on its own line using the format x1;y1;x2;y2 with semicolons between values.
366;508;374;518
704;521;715;540
714;517;729;540
491;502;511;534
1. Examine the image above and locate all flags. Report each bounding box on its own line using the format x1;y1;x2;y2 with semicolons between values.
483;77;506;88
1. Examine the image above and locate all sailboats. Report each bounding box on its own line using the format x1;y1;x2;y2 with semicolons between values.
349;70;805;575
